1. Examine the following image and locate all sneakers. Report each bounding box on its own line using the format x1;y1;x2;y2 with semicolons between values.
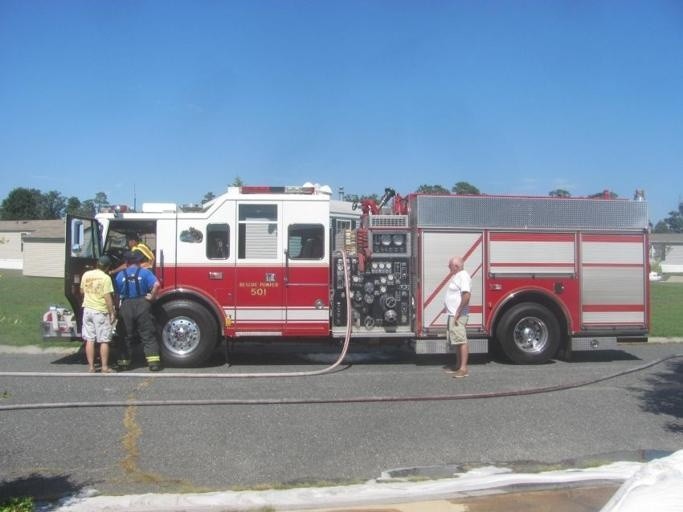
100;367;117;373
119;363;129;371
149;364;159;371
88;366;98;373
445;367;469;379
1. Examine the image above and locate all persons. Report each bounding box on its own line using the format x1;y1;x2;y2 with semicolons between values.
441;255;472;380
77;254;118;374
106;232;155;275
112;250;162;372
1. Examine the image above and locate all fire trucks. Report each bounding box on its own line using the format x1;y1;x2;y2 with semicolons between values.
60;183;652;368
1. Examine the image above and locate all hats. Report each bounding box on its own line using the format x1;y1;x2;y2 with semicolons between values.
97;256;114;267
